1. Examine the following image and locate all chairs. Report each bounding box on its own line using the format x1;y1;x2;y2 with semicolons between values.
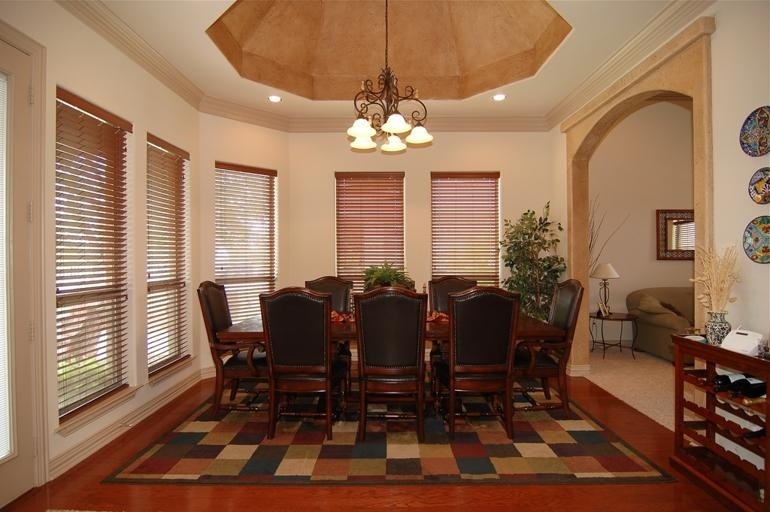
626;286;695;365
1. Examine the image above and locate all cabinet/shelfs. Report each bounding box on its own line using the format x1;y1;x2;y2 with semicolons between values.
668;332;770;512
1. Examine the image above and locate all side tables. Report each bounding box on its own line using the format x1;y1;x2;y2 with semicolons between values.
589;311;640;360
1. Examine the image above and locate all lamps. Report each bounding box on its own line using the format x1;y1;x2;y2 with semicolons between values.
347;0;434;153
589;262;621;317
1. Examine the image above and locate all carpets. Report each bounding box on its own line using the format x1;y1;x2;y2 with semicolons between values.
99;380;681;485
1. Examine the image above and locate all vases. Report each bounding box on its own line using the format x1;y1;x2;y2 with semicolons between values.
705;311;731;346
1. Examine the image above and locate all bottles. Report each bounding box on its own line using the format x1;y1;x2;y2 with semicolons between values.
707;373;766;399
